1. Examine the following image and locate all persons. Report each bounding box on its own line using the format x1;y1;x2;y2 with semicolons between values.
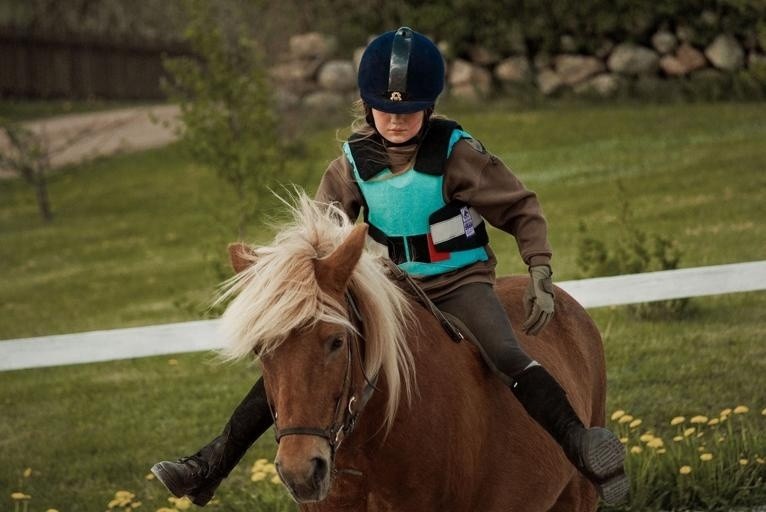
149;28;634;505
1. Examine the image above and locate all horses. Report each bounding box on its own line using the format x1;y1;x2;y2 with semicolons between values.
199;182;610;512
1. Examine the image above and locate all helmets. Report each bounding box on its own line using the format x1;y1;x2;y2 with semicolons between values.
359;27;444;114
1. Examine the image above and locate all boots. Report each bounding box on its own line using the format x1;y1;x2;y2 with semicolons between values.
510;365;630;504
150;375;275;506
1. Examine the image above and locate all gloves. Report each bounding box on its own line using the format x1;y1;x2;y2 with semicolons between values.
522;265;556;336
